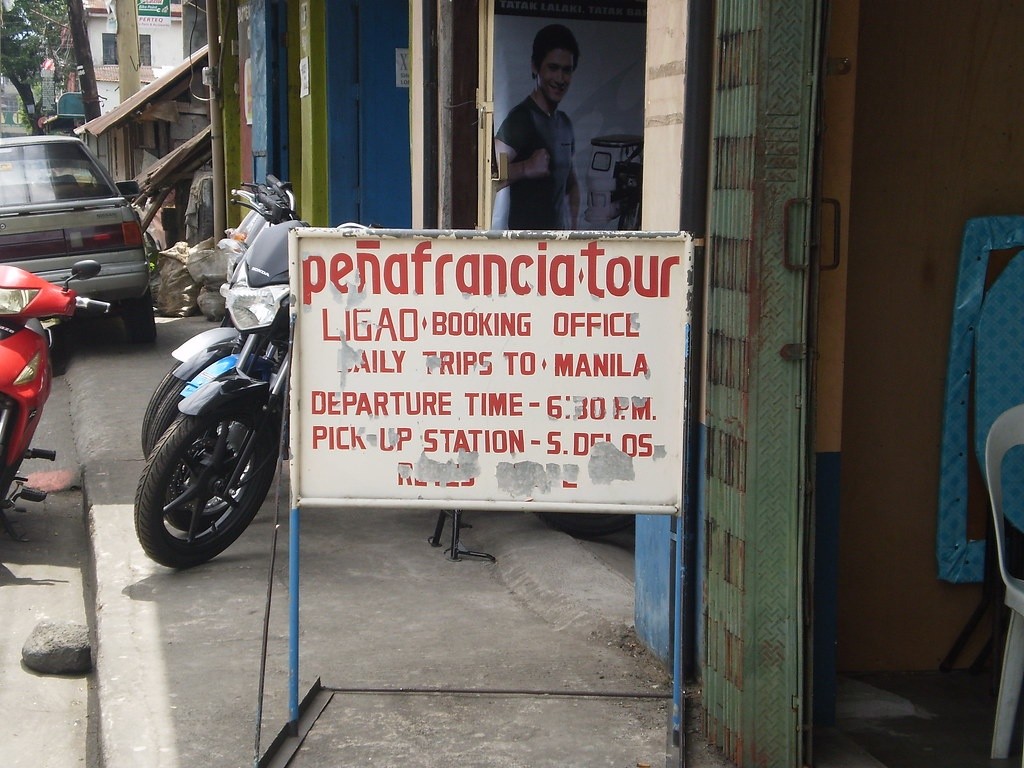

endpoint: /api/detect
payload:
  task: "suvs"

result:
[1,134,163,344]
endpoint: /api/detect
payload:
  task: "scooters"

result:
[119,177,645,573]
[0,259,113,520]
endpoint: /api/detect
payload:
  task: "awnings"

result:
[43,92,86,125]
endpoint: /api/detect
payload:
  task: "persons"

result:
[492,24,583,229]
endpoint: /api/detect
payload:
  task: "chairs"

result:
[38,174,79,198]
[986,400,1024,759]
